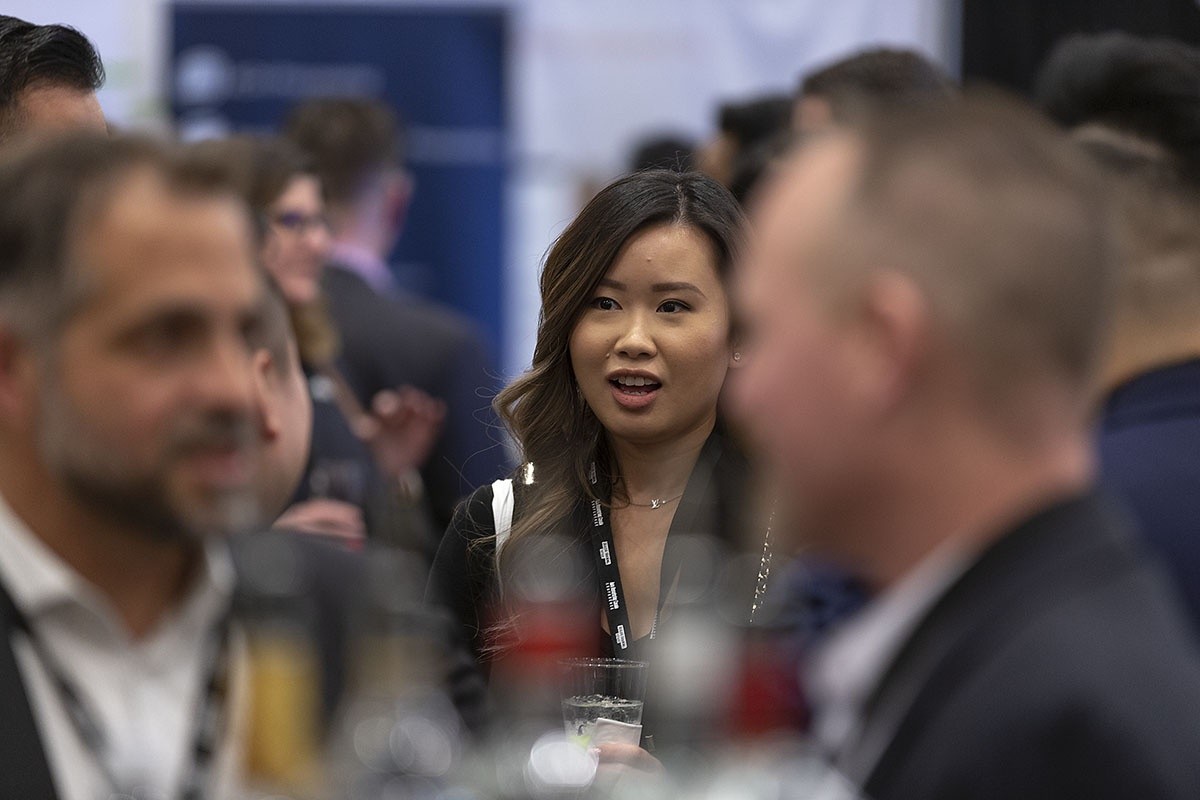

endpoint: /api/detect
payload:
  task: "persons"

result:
[0,15,1199,798]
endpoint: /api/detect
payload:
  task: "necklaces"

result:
[610,488,683,509]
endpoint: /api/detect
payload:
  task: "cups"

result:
[555,657,648,744]
